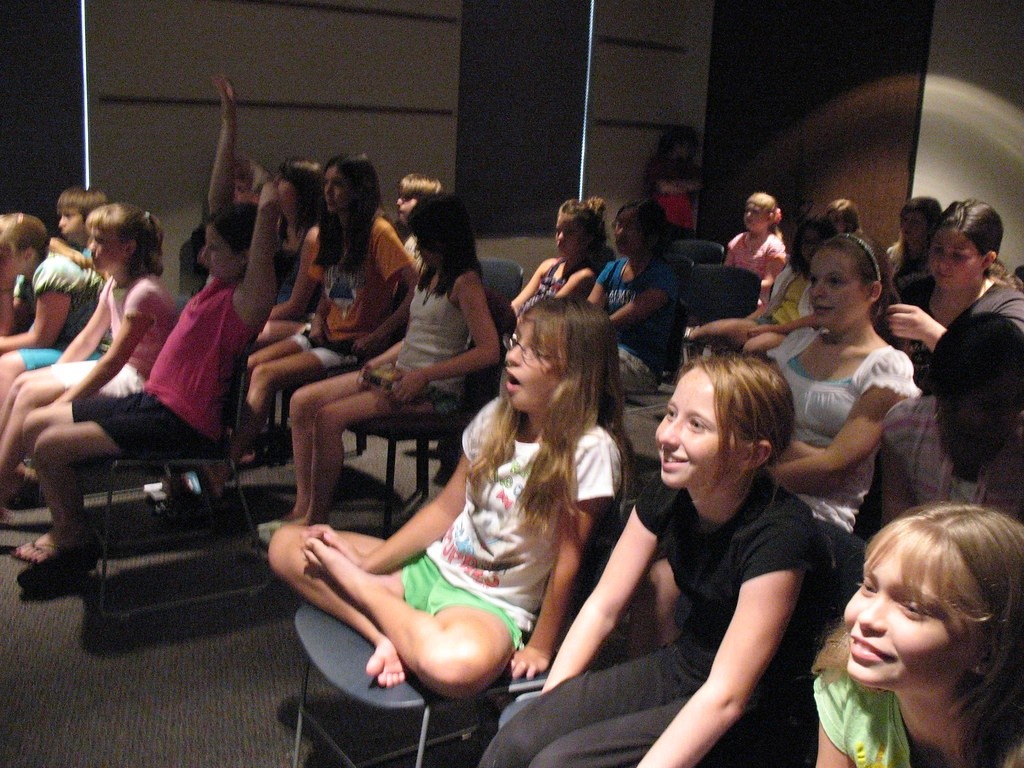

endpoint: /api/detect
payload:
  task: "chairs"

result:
[90,239,763,768]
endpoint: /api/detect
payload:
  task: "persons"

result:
[880,308,1024,531]
[885,199,1024,396]
[0,71,940,768]
[810,502,1024,768]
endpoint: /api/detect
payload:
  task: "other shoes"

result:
[256,519,332,547]
[11,467,43,507]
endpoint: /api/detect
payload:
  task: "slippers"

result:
[10,530,91,566]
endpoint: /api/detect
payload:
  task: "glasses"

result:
[502,332,552,360]
